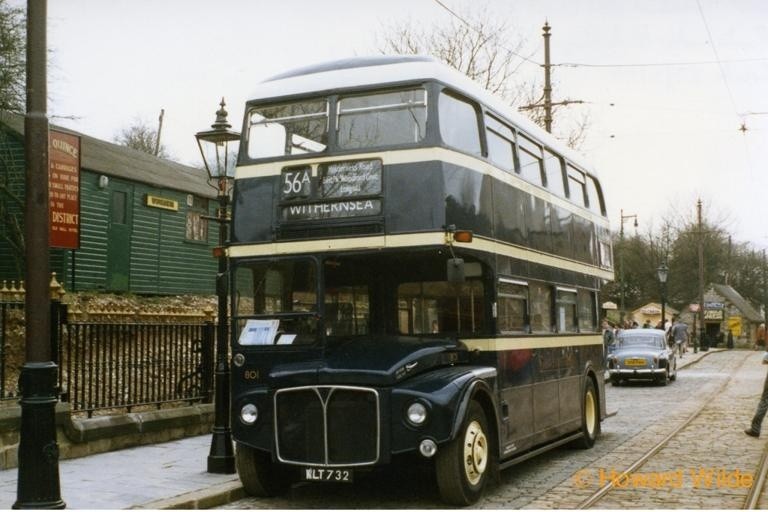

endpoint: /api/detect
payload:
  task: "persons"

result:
[753,322,765,352]
[601,316,691,362]
[742,352,768,438]
[431,321,438,333]
[726,328,734,348]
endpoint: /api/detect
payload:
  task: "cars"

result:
[608,329,677,387]
[643,319,690,355]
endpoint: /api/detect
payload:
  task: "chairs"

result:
[308,304,352,336]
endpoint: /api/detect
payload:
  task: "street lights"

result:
[619,212,638,328]
[196,96,243,475]
[655,263,669,332]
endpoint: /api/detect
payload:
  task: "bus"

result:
[228,54,620,505]
